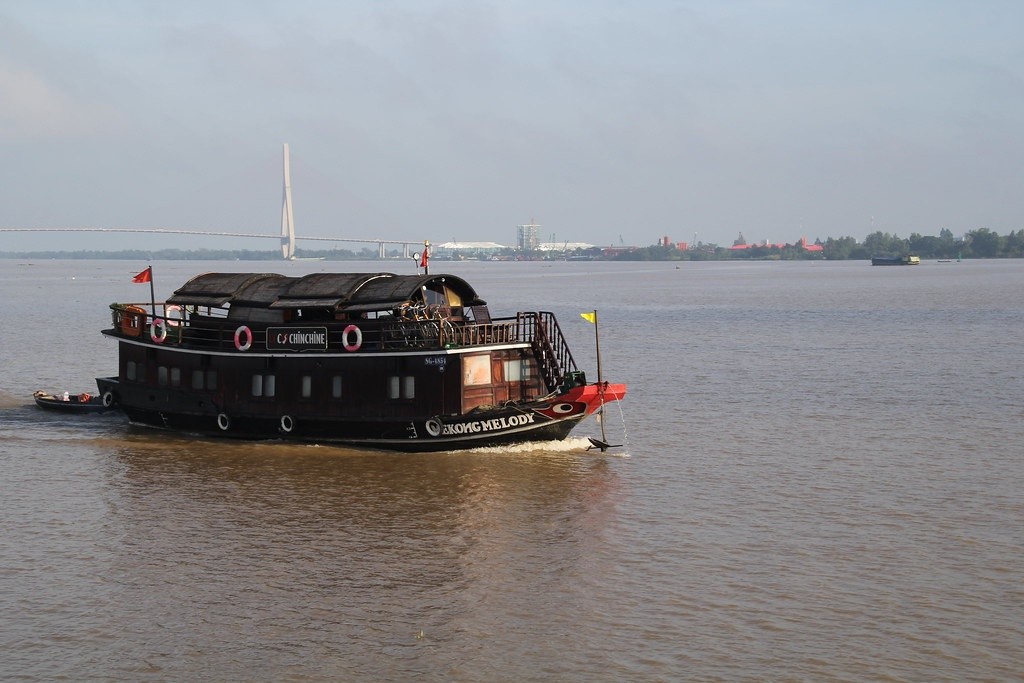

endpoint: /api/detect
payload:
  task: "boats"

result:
[292,256,326,262]
[102,269,627,451]
[938,260,952,262]
[34,392,107,412]
[870,255,921,265]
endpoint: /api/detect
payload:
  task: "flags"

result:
[420,248,427,267]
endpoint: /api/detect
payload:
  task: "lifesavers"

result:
[217,413,229,431]
[120,306,148,337]
[102,392,114,407]
[423,417,443,437]
[165,306,185,327]
[150,319,167,343]
[233,326,252,351]
[279,415,294,432]
[341,325,362,352]
